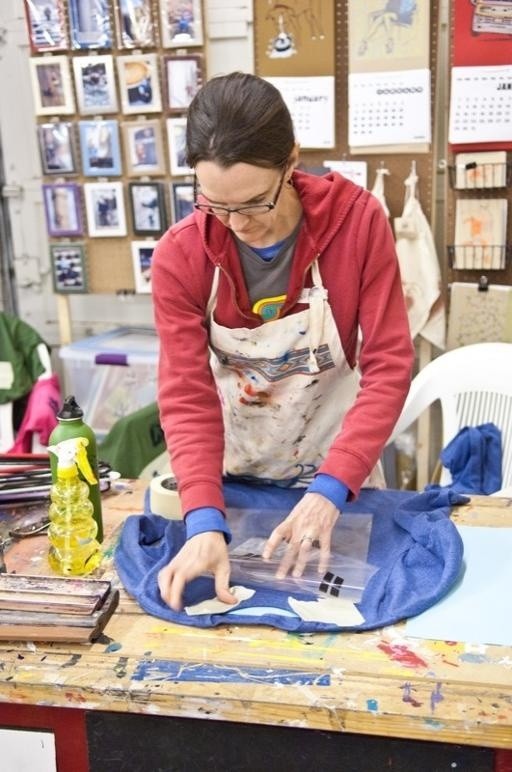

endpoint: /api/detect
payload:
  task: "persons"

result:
[357,0,417,56]
[148,71,418,613]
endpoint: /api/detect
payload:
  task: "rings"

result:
[300,535,315,544]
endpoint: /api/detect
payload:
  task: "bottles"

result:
[46,394,105,545]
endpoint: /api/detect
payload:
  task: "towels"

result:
[370,161,398,218]
[394,161,442,341]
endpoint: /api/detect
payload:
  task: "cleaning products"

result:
[48,438,102,575]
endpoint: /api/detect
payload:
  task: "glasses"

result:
[191,167,285,220]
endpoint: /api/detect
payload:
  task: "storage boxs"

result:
[59,326,161,444]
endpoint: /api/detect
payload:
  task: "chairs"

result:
[367,341,511,498]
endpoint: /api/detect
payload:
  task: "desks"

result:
[1,476,512,771]
